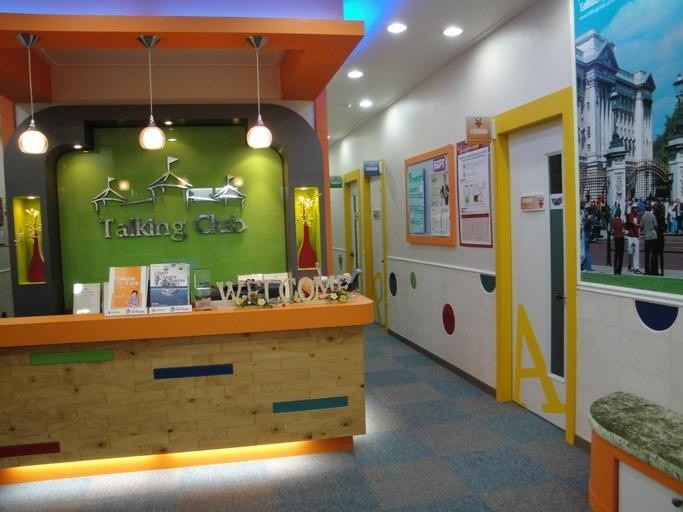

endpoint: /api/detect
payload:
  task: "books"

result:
[106,265,148,309]
[149,263,190,307]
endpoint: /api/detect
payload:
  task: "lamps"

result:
[246,35,273,149]
[14,33,48,155]
[138,35,165,150]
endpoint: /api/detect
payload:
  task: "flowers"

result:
[326,289,353,303]
[295,192,322,226]
[13,209,42,246]
[235,292,274,308]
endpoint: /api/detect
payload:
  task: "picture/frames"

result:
[569,0,683,308]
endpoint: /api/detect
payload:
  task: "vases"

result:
[299,228,317,268]
[28,238,44,282]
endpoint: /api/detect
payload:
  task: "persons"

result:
[126,289,138,307]
[579,186,682,278]
[438,173,448,205]
